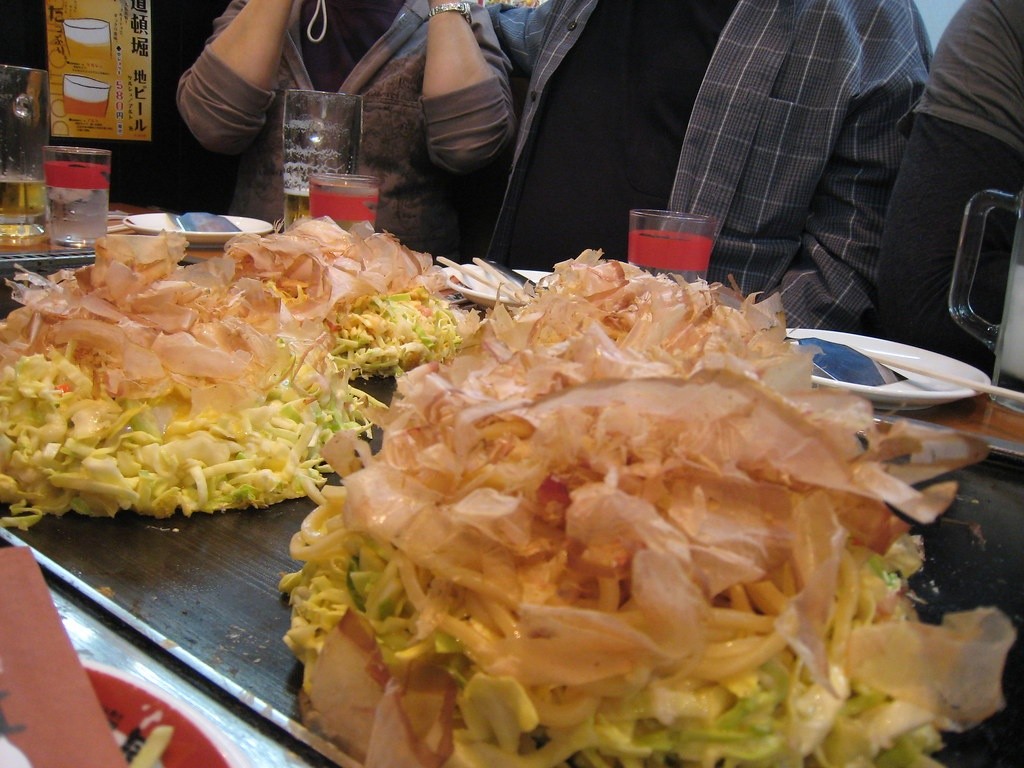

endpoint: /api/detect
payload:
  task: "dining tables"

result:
[0,195,1024,768]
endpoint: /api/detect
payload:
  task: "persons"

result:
[175,0,1024,381]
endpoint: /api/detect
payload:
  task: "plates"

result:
[123,212,273,248]
[447,270,553,310]
[783,328,991,411]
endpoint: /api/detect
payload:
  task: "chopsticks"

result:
[109,214,127,220]
[869,352,1024,403]
[436,256,527,302]
[108,223,129,234]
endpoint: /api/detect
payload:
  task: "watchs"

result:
[428,2,472,23]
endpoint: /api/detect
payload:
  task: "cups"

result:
[308,173,380,233]
[628,208,722,282]
[948,187,1024,418]
[0,63,51,244]
[283,90,364,232]
[43,145,110,251]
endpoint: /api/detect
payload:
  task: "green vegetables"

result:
[0,282,948,768]
